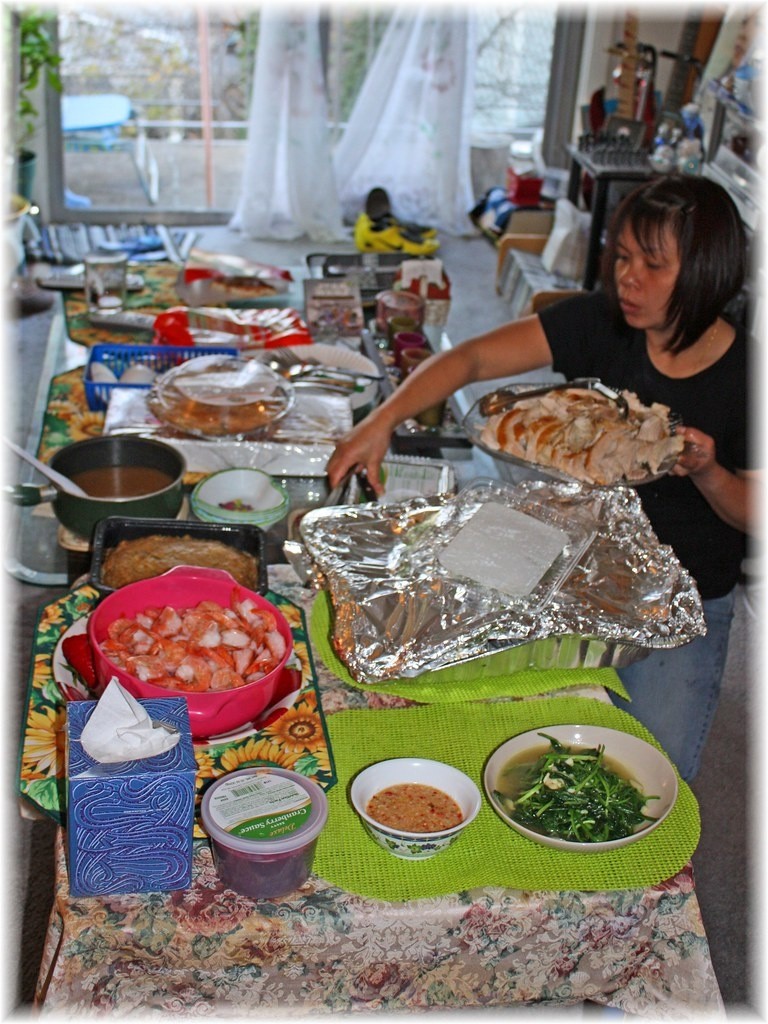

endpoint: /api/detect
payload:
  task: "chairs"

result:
[61,93,161,206]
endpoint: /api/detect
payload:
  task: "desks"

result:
[564,140,677,290]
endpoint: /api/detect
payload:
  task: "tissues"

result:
[62,674,198,899]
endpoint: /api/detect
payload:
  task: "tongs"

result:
[479,377,628,418]
[319,468,379,509]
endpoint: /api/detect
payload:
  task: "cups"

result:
[417,397,446,426]
[388,315,421,350]
[85,248,129,310]
[394,332,427,367]
[400,348,431,385]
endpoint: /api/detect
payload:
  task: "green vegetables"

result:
[491,732,664,843]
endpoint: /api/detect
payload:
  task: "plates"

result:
[53,610,302,746]
[462,383,684,488]
[484,724,678,852]
[254,346,378,410]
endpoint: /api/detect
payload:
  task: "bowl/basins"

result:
[147,361,294,442]
[87,566,293,737]
[87,516,268,608]
[351,758,481,861]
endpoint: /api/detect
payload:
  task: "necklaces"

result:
[647,315,720,372]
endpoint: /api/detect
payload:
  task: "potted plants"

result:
[13,10,65,200]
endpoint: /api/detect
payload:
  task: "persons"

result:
[325,173,765,785]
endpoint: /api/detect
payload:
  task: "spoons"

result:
[264,359,352,399]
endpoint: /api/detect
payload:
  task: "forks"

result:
[265,343,385,385]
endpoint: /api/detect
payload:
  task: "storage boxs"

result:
[495,211,553,264]
[495,249,581,317]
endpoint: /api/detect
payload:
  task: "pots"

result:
[5,435,186,544]
